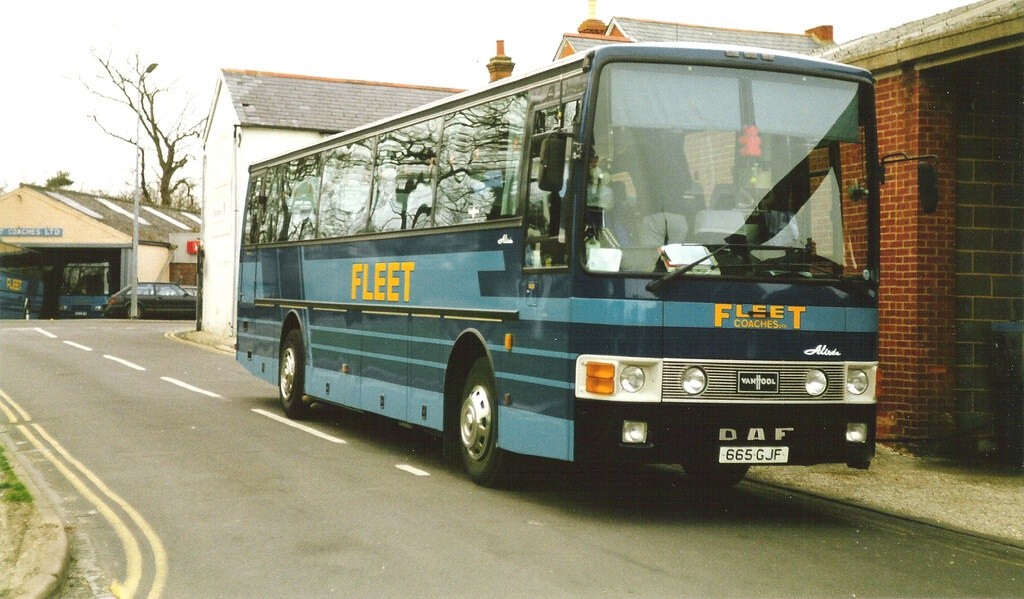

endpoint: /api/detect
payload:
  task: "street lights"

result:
[130,64,159,319]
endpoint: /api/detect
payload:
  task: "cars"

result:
[100,280,197,320]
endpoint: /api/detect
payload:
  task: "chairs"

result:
[605,161,801,248]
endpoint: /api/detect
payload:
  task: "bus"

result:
[54,259,112,318]
[0,239,46,322]
[232,39,881,512]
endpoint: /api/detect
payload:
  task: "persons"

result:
[23,294,31,321]
[586,146,798,243]
